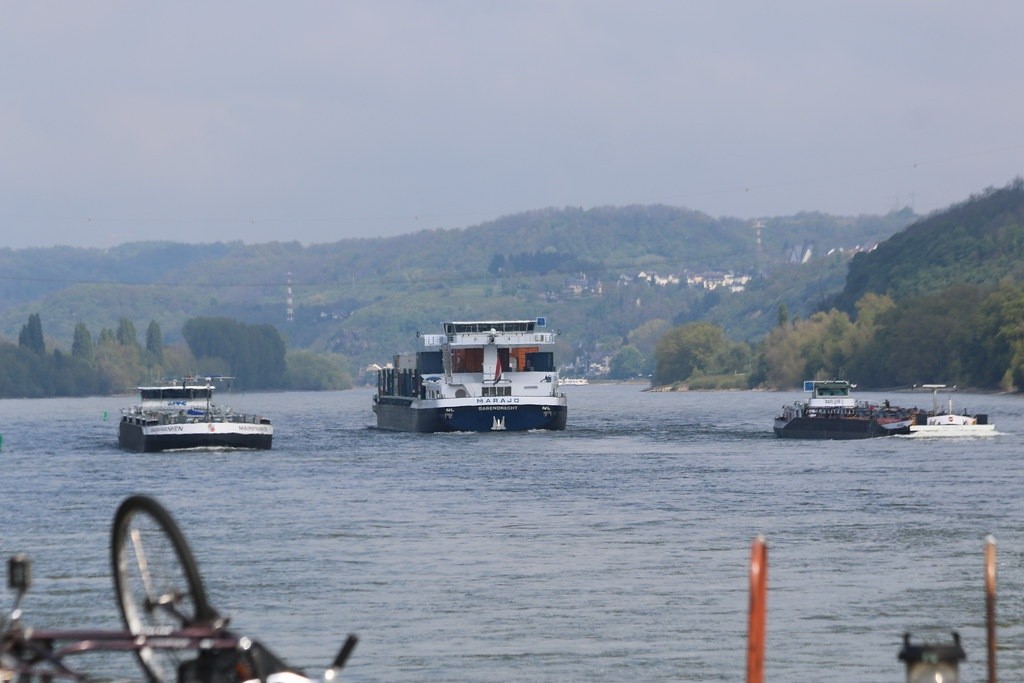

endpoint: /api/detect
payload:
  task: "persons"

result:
[882,399,890,407]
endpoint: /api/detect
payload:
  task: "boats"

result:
[883,397,996,439]
[361,315,568,434]
[116,367,274,451]
[771,370,995,439]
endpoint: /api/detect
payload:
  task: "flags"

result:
[493,356,502,385]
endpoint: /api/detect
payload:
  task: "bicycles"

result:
[0,492,360,683]
